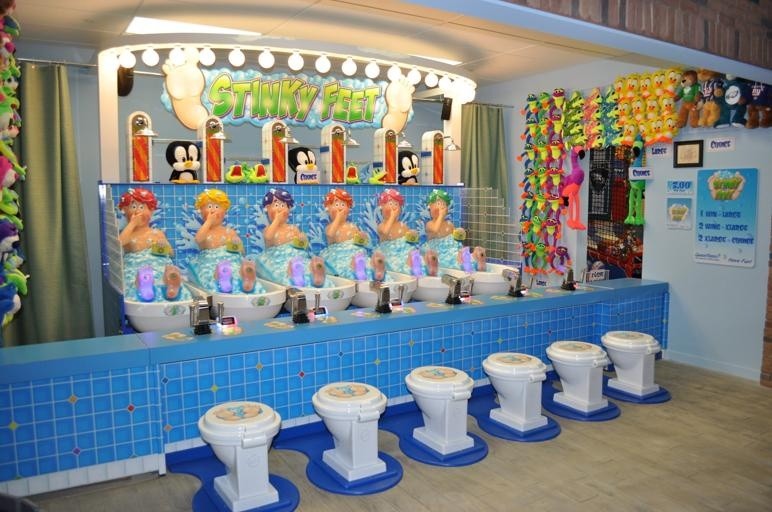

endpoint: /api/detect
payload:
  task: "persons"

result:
[261,187,325,288]
[321,187,386,281]
[426,188,486,274]
[115,187,181,301]
[376,188,438,277]
[194,188,257,293]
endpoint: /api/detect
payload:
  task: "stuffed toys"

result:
[368,166,387,185]
[165,141,201,184]
[398,150,421,185]
[0,0,30,330]
[515,66,772,274]
[288,147,322,184]
[345,161,362,185]
[225,161,268,184]
[515,67,772,275]
